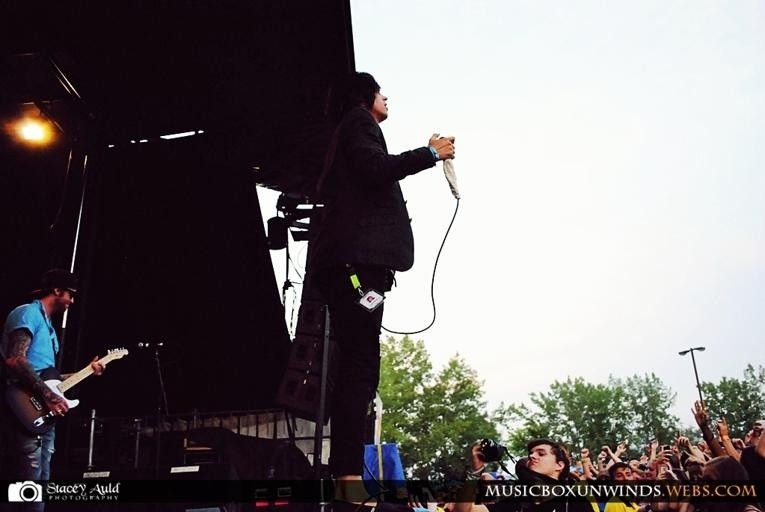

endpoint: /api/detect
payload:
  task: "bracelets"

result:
[426,144,440,162]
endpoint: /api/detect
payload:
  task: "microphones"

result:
[439,137,461,199]
[138,342,163,348]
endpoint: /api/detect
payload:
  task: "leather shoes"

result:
[334,499,415,512]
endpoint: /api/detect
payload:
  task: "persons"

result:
[406,399,765,511]
[311,72,456,512]
[2,267,107,512]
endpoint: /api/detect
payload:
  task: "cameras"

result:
[478,439,506,462]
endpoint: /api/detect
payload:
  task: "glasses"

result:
[67,288,77,298]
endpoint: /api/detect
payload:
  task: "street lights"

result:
[676,345,705,411]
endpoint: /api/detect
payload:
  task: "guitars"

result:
[9,347,128,435]
